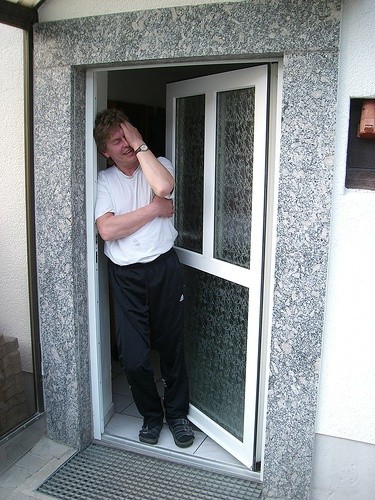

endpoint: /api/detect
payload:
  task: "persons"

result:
[93,108,195,448]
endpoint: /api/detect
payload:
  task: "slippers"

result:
[139,419,163,444]
[169,419,195,447]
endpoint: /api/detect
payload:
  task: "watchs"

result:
[133,144,151,155]
